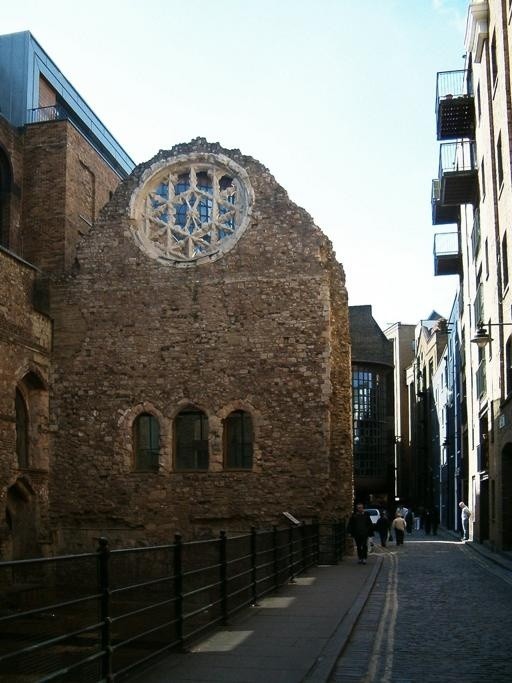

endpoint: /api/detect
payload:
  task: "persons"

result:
[375,513,391,547]
[459,502,471,540]
[423,512,432,535]
[392,512,407,546]
[405,511,413,536]
[430,507,440,536]
[347,503,375,566]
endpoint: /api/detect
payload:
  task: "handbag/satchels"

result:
[389,536,393,541]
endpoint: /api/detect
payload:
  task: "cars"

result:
[364,508,381,524]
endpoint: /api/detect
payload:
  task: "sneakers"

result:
[409,533,411,536]
[358,559,367,564]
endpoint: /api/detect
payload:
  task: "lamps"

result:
[467,319,512,349]
